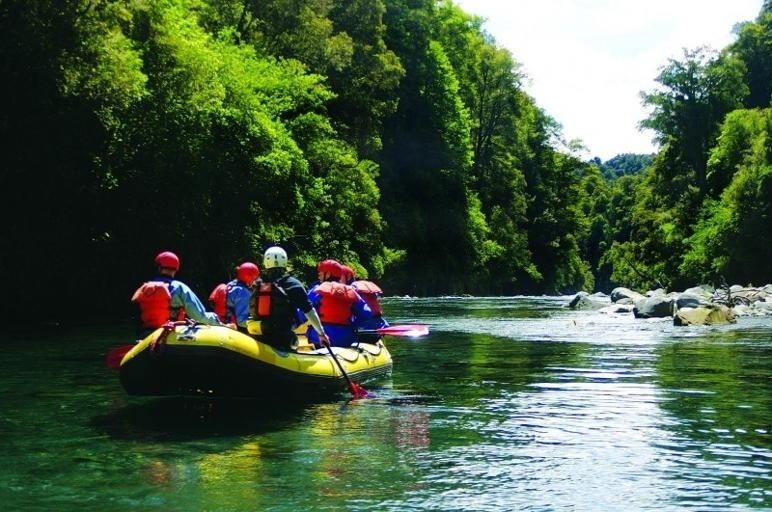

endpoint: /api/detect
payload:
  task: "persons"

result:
[132,246,388,353]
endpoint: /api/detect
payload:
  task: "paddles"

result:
[108,344,134,368]
[324,340,367,400]
[355,324,429,338]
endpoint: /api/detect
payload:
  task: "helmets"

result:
[340,264,355,283]
[262,245,289,269]
[318,258,343,279]
[237,261,261,285]
[154,250,181,271]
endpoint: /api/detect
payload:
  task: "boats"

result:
[119,318,393,399]
